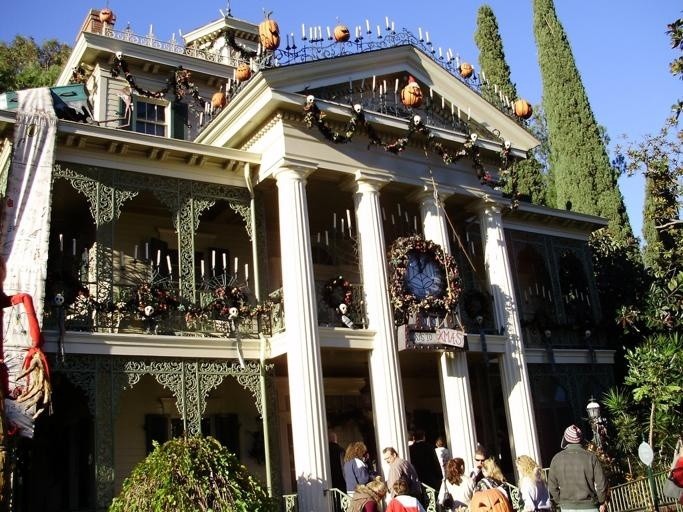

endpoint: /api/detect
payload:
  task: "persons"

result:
[343,439,377,512]
[406,430,551,511]
[546,425,606,511]
[382,446,422,494]
[383,479,425,511]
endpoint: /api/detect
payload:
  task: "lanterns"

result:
[98,8,113,24]
[458,63,472,79]
[514,98,532,120]
[210,93,224,108]
[233,63,250,82]
[258,19,280,52]
[334,26,350,42]
[400,82,421,107]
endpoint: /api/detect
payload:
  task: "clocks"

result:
[406,253,445,301]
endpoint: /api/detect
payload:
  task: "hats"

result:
[560,425,584,449]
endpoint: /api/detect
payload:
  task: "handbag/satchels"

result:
[443,492,454,508]
[504,481,525,510]
